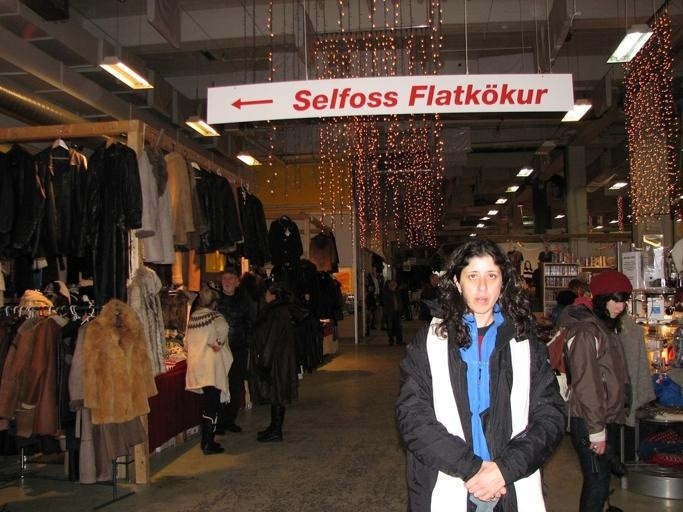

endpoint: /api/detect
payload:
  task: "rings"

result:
[594,446,597,449]
[491,497,495,500]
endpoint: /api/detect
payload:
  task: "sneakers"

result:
[227,422,243,433]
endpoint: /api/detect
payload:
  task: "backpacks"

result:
[546,328,603,402]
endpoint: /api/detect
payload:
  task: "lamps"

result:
[465,165,535,238]
[186,61,221,137]
[561,41,592,123]
[236,153,262,166]
[606,0,654,64]
[97,1,155,92]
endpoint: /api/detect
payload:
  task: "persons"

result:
[251,283,295,443]
[366,244,587,345]
[564,270,633,512]
[184,288,233,455]
[393,239,568,512]
[190,267,257,436]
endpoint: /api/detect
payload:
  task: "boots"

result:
[257,405,285,442]
[200,414,225,455]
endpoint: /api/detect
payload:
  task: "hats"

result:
[589,271,633,297]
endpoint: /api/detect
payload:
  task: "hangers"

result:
[0,301,97,326]
[49,129,71,160]
[105,135,116,150]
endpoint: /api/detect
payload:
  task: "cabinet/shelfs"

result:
[632,287,680,375]
[538,262,614,313]
